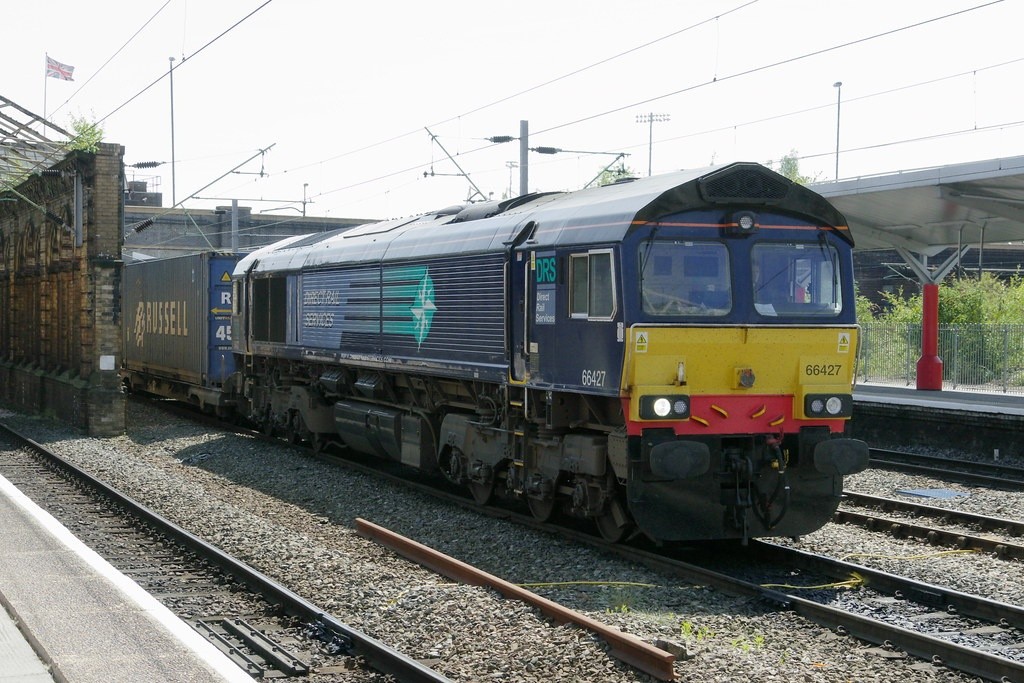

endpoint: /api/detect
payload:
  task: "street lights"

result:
[169,56,177,207]
[832,82,842,185]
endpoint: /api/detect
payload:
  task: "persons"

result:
[727,260,767,309]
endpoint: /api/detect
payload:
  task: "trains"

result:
[110,158,872,548]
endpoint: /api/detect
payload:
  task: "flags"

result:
[47,56,75,81]
